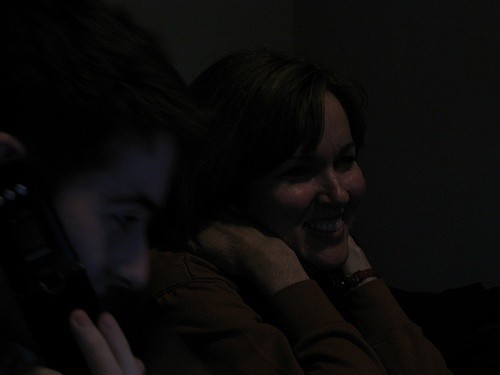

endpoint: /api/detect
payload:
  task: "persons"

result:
[113,48,453,375]
[0,0,213,375]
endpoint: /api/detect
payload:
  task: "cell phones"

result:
[0,160,103,375]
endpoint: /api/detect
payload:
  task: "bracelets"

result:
[330,268,382,298]
